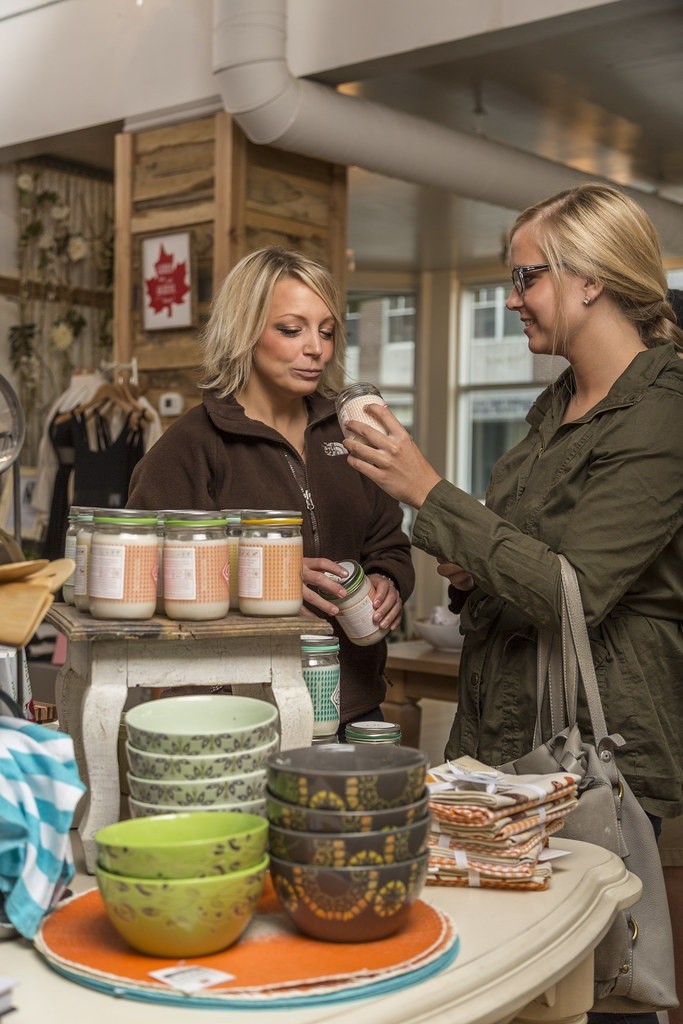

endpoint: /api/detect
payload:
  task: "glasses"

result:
[511,261,566,297]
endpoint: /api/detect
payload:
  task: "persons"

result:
[342,182,683,1024]
[123,249,416,743]
[31,367,111,602]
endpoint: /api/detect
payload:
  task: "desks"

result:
[0,838,644,1024]
[379,640,460,753]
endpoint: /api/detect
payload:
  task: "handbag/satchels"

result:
[470,553,681,1014]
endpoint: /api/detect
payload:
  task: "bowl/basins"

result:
[93,695,431,957]
[413,616,465,653]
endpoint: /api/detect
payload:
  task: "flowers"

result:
[12,163,114,466]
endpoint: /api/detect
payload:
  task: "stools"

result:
[47,604,335,877]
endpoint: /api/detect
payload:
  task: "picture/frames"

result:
[134,230,199,331]
